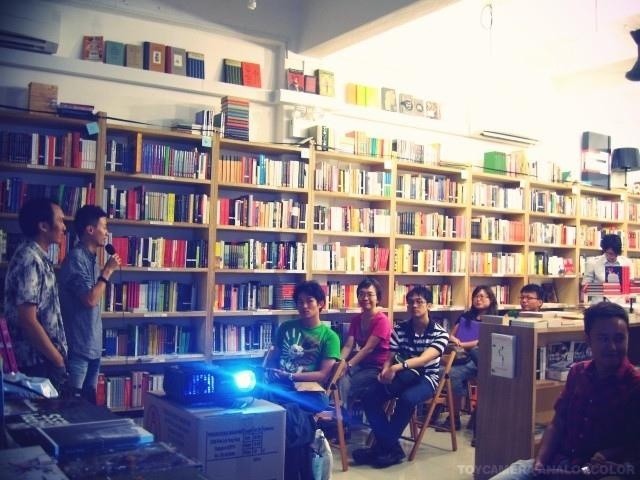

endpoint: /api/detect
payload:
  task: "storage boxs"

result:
[141,388,287,480]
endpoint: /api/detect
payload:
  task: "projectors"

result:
[163,362,258,406]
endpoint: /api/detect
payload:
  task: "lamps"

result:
[610,147,640,190]
[247,0,257,10]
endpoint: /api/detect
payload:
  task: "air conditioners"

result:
[0,0,62,55]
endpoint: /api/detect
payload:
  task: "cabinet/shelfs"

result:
[393,158,470,326]
[0,108,107,279]
[310,151,393,327]
[626,191,640,280]
[579,182,626,278]
[469,169,529,312]
[472,321,640,480]
[198,137,310,363]
[528,177,579,311]
[95,118,221,424]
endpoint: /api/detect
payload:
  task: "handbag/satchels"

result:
[310,428,333,479]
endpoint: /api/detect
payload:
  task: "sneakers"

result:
[352,447,378,466]
[376,445,406,469]
[436,417,462,432]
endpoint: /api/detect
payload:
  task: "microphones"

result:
[105,244,115,255]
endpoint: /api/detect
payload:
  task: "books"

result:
[0,35,640,479]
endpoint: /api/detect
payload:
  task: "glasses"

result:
[518,296,541,301]
[406,299,428,307]
[357,292,376,300]
[605,248,616,256]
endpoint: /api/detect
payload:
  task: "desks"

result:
[1,383,216,480]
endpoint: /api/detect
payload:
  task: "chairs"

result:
[311,358,356,472]
[365,350,457,461]
